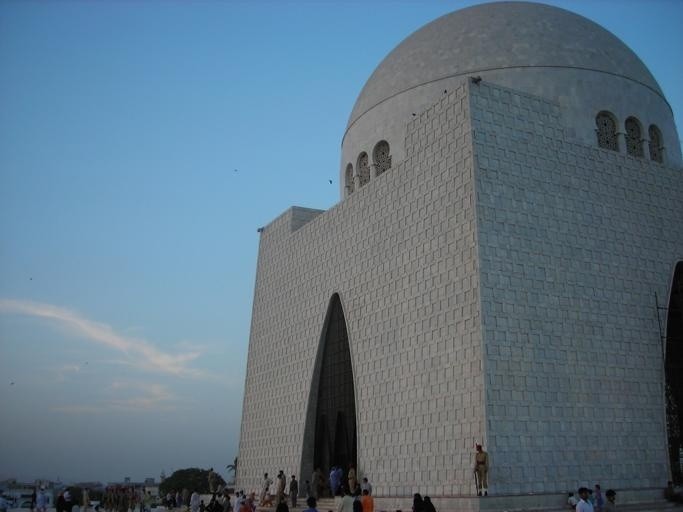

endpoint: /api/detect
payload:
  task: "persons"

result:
[565,483,616,512]
[412,493,435,511]
[1,478,154,511]
[472,442,489,496]
[160,463,374,511]
[662,481,682,506]
